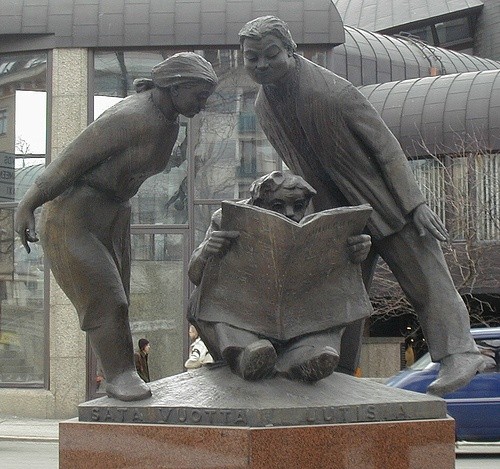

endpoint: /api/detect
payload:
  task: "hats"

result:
[138,338,149,350]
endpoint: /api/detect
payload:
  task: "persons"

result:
[14,49,218,401]
[240,17,489,395]
[95,369,105,392]
[185,325,214,373]
[133,337,152,384]
[184,171,346,384]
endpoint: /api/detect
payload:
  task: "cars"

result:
[381,327,500,443]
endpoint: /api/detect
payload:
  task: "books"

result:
[198,200,375,343]
